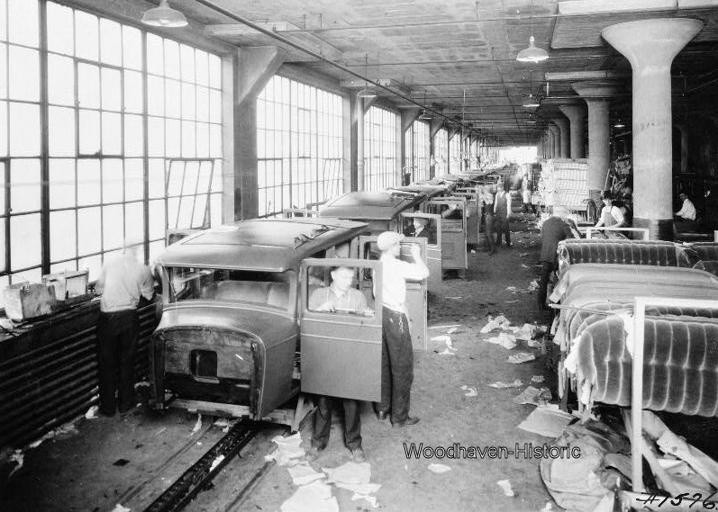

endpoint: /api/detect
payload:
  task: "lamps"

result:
[517,0,550,62]
[141,0,188,28]
[614,118,625,128]
[356,53,376,97]
[527,113,536,123]
[522,68,540,107]
[419,90,431,120]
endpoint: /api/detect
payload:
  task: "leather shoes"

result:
[377,409,419,429]
[303,444,324,463]
[351,446,367,463]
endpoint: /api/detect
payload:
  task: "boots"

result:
[474,235,497,255]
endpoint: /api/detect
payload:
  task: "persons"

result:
[92,244,154,418]
[475,166,535,256]
[371,229,431,429]
[305,264,375,462]
[672,189,698,232]
[538,204,574,310]
[442,201,462,280]
[594,191,625,239]
[408,210,433,238]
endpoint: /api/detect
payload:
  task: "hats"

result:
[376,229,406,251]
[599,189,616,201]
